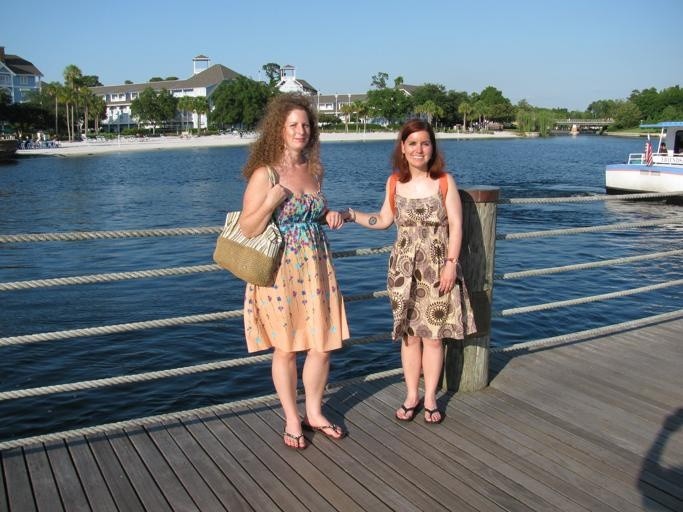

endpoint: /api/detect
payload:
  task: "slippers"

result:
[396,405,443,424]
[282,422,346,449]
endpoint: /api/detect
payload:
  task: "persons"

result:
[24,137,31,149]
[660,143,667,155]
[236,90,344,449]
[342,119,477,425]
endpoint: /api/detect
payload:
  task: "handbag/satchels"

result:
[212,211,283,286]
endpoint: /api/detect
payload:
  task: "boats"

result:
[605,122,683,194]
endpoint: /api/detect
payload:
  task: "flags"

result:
[645,134,652,167]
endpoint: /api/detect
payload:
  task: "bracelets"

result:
[443,257,458,264]
[353,210,356,221]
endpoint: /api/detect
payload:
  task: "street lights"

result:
[317,90,351,125]
